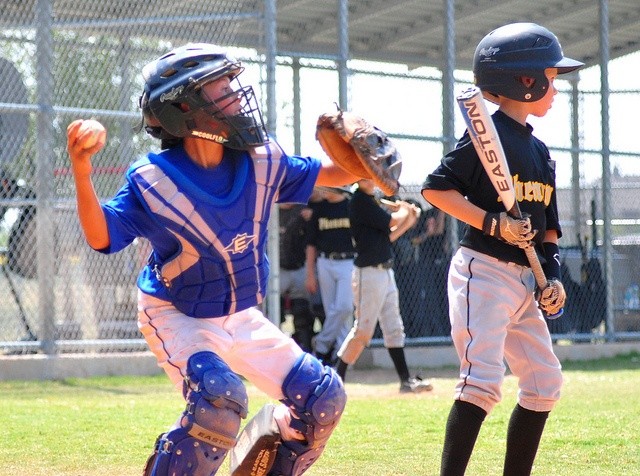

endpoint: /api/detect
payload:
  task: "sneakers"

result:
[400,377,432,393]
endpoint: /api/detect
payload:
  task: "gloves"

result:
[535,279,567,315]
[482,211,540,249]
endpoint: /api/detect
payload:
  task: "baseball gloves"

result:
[315,101,403,197]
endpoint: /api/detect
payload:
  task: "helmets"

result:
[136,42,273,152]
[472,22,586,106]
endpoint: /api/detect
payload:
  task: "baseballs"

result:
[77,120,106,149]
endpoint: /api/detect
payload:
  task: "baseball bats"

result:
[456,86,564,320]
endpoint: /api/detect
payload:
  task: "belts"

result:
[326,252,354,260]
[372,262,394,270]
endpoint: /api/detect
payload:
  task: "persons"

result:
[66,42,404,475]
[393,197,434,338]
[419,21,587,476]
[332,180,434,394]
[547,258,579,343]
[574,258,607,343]
[279,202,325,353]
[305,186,356,368]
[420,205,453,338]
